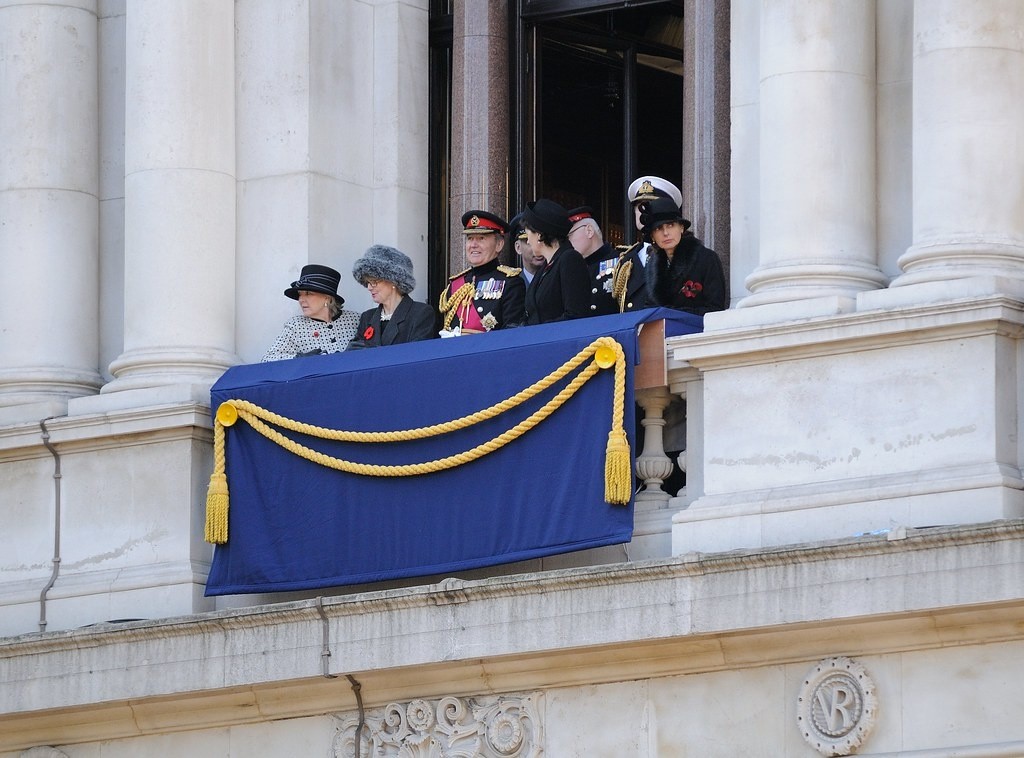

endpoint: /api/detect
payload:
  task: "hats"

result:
[523,199,573,236]
[461,210,512,238]
[567,206,594,225]
[284,265,345,304]
[638,197,691,243]
[509,212,528,240]
[352,244,416,295]
[628,176,683,210]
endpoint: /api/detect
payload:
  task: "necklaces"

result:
[381,308,393,321]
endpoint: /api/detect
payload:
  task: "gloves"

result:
[438,325,461,339]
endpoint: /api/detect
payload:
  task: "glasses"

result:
[363,279,383,287]
[567,224,587,238]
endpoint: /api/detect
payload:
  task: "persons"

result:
[434,210,526,340]
[260,265,363,364]
[345,245,436,353]
[518,199,591,326]
[568,175,728,318]
[510,212,546,289]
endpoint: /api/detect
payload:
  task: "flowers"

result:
[679,280,702,297]
